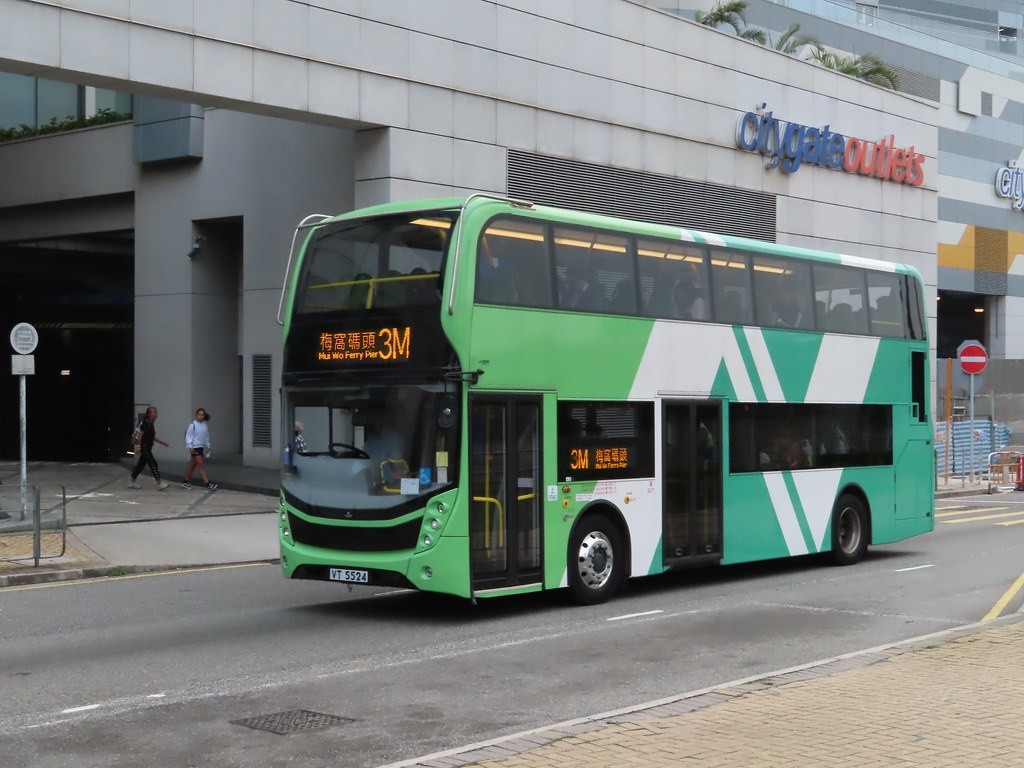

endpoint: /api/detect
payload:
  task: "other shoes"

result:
[128,482,142,488]
[157,482,170,491]
[274,505,278,512]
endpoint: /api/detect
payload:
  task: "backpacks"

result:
[184,423,195,445]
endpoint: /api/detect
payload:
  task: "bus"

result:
[269,192,937,606]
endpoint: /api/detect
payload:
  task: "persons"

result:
[179,408,218,490]
[128,406,170,491]
[295,421,308,452]
[354,416,850,545]
[440,230,834,333]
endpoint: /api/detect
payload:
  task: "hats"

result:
[778,273,807,290]
[295,422,303,431]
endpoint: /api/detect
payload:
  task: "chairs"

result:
[378,270,402,306]
[348,272,375,306]
[717,290,743,320]
[831,303,853,332]
[406,267,434,304]
[805,301,826,330]
[649,285,678,316]
[480,265,524,305]
[611,277,645,314]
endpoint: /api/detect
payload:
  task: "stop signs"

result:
[959,343,989,376]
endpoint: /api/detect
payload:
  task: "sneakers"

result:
[206,483,217,490]
[181,479,192,489]
